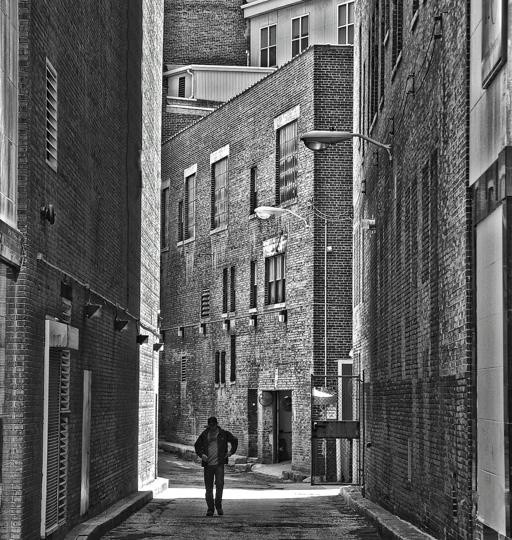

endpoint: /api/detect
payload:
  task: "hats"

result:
[208,417,217,425]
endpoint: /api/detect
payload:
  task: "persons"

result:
[195,417,238,516]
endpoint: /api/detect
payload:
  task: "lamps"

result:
[301,130,394,162]
[254,205,309,229]
[83,291,165,353]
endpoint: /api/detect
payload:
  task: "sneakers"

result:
[206,506,223,516]
